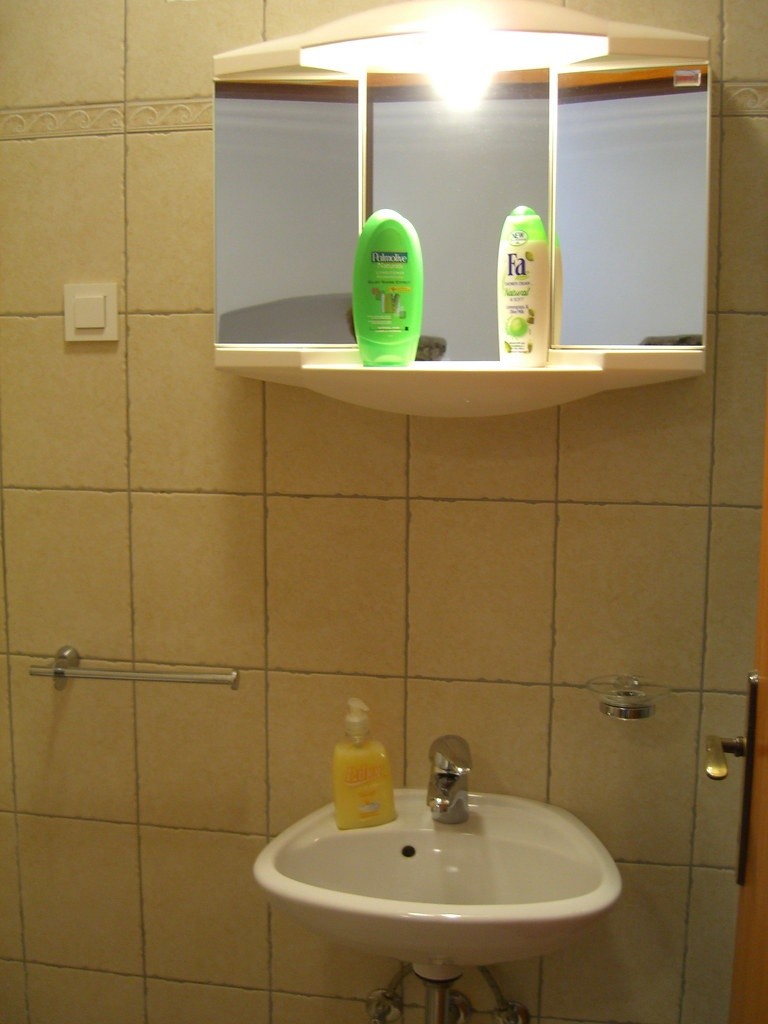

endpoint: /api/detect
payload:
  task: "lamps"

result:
[298,0,610,113]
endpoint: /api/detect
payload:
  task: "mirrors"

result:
[210,21,710,418]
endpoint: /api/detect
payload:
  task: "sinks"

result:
[253,789,622,963]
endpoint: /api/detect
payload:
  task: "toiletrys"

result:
[495,205,551,367]
[331,697,396,830]
[352,207,423,367]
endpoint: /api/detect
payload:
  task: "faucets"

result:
[427,733,470,822]
